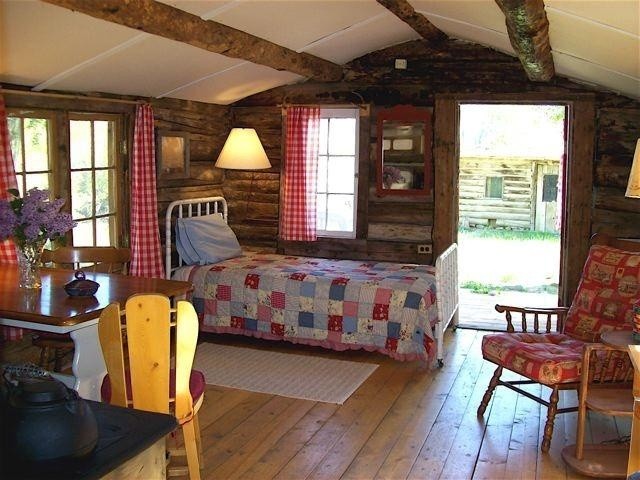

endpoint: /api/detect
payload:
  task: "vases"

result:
[384,183,393,190]
[13,238,50,288]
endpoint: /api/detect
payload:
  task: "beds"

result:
[166,196,459,368]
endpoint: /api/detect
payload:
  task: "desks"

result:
[0,262,194,403]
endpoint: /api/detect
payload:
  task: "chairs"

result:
[98,293,207,480]
[477,232,640,453]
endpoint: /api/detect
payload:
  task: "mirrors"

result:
[376,105,431,197]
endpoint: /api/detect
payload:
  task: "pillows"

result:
[175,214,242,265]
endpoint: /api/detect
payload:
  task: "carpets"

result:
[193,341,379,405]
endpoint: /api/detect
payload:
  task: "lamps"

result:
[625,138,640,198]
[214,128,272,170]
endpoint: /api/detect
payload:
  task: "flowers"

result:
[0,187,78,273]
[382,164,403,186]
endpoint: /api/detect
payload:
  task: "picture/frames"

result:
[156,131,190,181]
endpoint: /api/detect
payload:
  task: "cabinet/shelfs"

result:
[561,330,640,480]
[627,344,640,480]
[0,401,179,480]
